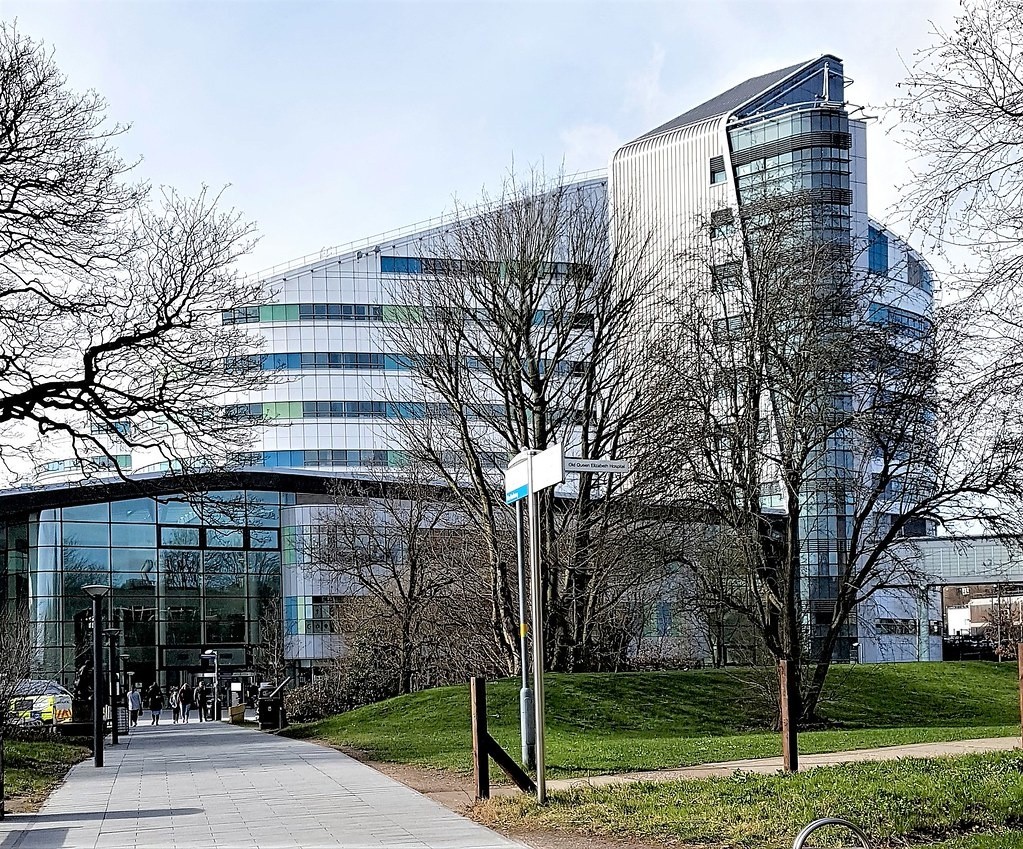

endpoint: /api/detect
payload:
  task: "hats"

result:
[199,681,205,686]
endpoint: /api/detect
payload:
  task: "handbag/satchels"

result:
[139,705,143,716]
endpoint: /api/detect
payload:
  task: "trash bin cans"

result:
[258,687,282,730]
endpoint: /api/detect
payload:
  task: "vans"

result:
[3,679,74,725]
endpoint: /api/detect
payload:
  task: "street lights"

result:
[119,653,130,708]
[80,584,111,768]
[103,628,123,745]
[127,671,135,692]
[992,583,1001,663]
[204,649,218,721]
[507,445,544,771]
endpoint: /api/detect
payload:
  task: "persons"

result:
[127,685,141,727]
[147,681,207,725]
